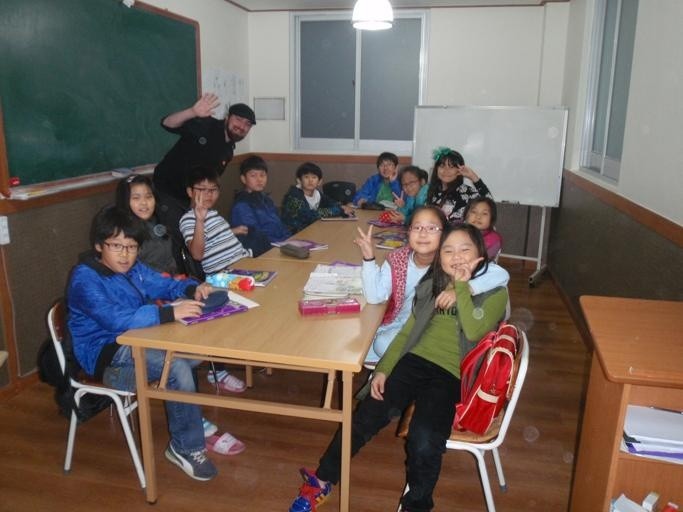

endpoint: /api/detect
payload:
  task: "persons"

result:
[421,149,494,225]
[386,165,430,228]
[229,154,293,257]
[348,151,403,212]
[351,204,510,360]
[114,172,246,438]
[278,161,356,232]
[152,89,257,207]
[63,202,216,482]
[288,219,510,511]
[176,166,250,275]
[458,195,502,262]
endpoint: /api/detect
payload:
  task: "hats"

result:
[229,103,256,124]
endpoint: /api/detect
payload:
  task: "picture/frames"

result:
[253,96,285,121]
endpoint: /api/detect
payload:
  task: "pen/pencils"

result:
[162,300,184,308]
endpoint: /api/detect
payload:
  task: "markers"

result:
[501,200,520,205]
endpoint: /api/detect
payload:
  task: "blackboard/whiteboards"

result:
[0,0,202,198]
[411,105,568,208]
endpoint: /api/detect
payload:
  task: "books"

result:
[318,216,359,222]
[299,263,364,295]
[326,260,362,267]
[268,237,329,252]
[373,237,403,251]
[208,267,278,288]
[156,288,261,327]
[618,404,683,467]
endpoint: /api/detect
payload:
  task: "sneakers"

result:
[289,468,332,512]
[164,439,217,481]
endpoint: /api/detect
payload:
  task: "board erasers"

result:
[111,167,131,178]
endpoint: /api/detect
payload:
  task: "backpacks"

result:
[453,321,520,435]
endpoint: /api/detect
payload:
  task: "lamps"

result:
[351,1,392,30]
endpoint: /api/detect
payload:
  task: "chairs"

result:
[44,298,159,489]
[395,330,528,511]
[176,236,207,279]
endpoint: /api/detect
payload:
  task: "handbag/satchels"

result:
[36,337,110,423]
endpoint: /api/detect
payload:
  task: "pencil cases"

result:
[298,298,360,316]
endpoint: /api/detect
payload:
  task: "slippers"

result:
[204,432,246,456]
[211,375,247,393]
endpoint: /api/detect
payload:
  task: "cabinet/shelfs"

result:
[565,294,682,510]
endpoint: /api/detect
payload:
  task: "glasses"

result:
[193,187,220,195]
[409,224,442,233]
[103,241,140,253]
[401,180,418,188]
